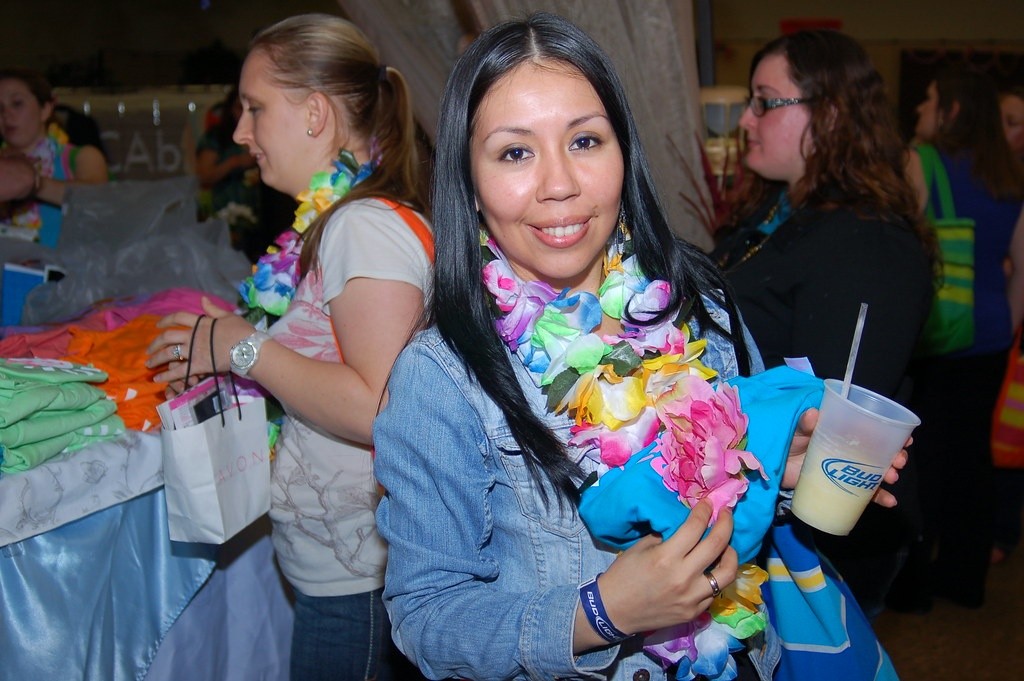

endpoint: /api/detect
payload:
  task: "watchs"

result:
[229,330,273,382]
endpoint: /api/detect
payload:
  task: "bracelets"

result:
[577,572,637,644]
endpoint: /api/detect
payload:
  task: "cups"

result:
[790,378,921,536]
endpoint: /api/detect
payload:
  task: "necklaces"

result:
[238,149,384,462]
[472,221,771,526]
[1,124,69,233]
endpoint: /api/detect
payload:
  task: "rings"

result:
[705,572,720,597]
[173,345,182,360]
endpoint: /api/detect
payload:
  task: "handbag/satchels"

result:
[159,314,272,546]
[914,145,977,347]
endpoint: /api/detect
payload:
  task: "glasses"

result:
[744,94,817,117]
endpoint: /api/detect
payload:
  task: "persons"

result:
[180,83,299,263]
[704,28,1024,635]
[0,69,109,253]
[147,14,438,680]
[372,15,912,681]
